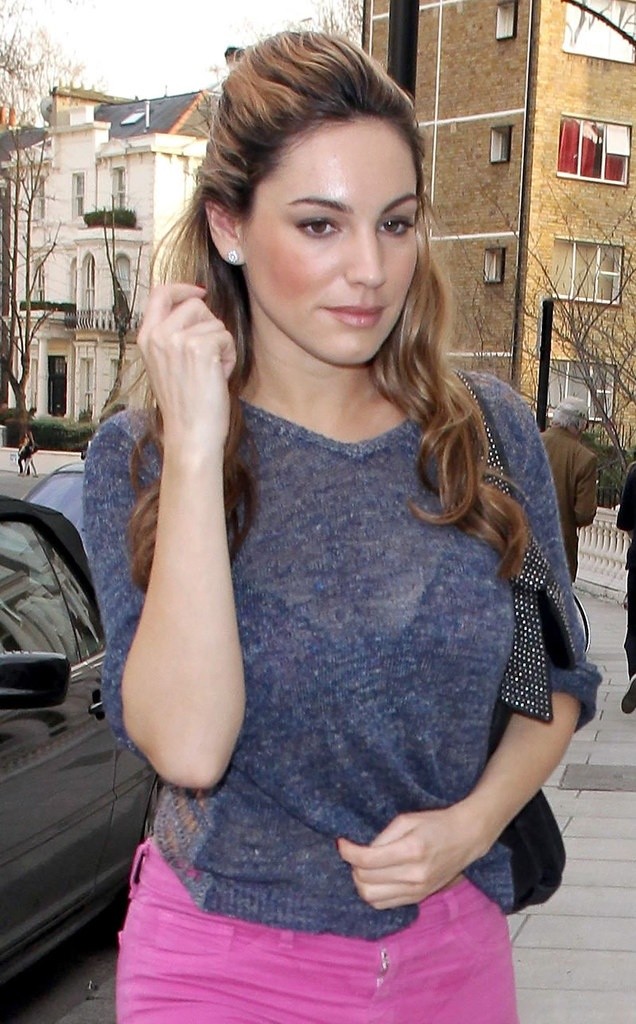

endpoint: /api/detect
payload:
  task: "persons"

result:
[539,396,598,582]
[84,32,602,1024]
[17,425,38,476]
[616,462,636,713]
[81,430,95,460]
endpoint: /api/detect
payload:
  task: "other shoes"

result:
[621,674,636,714]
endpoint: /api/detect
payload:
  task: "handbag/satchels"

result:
[453,369,576,908]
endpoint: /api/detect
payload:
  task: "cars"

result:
[0,456,162,987]
